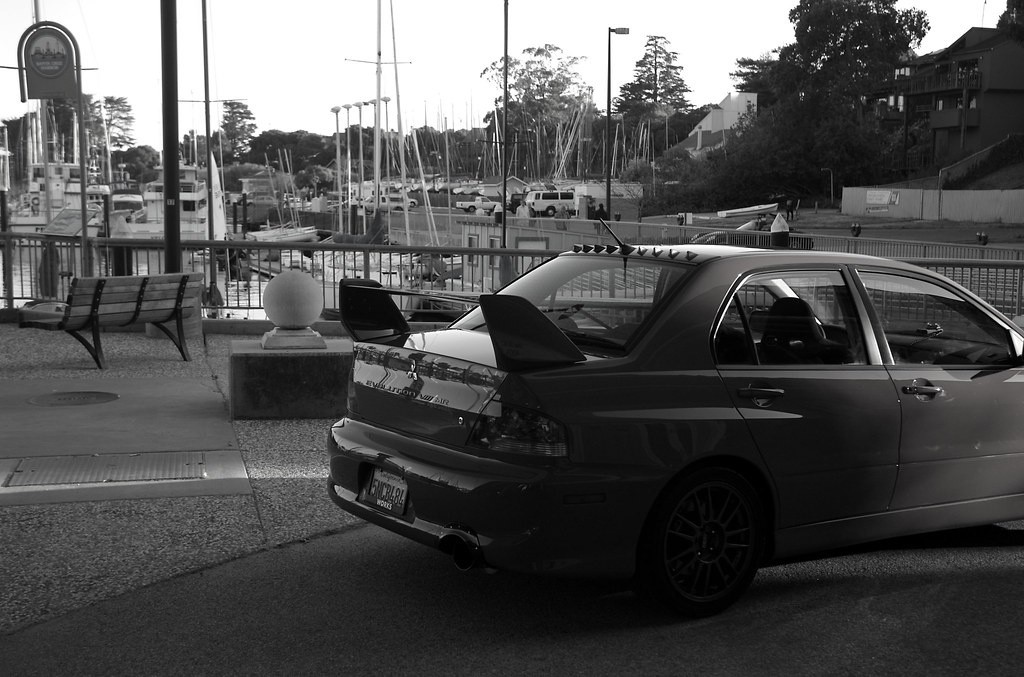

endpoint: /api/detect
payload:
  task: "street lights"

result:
[822,166,835,206]
[606,27,631,221]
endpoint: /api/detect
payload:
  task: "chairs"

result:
[19,271,204,370]
[716,329,753,364]
[760,297,840,366]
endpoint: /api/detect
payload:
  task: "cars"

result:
[390,194,418,208]
[360,195,411,214]
[284,193,302,203]
[323,242,1023,606]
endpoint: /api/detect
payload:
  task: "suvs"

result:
[510,193,527,214]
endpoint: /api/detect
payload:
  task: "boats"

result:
[717,202,779,218]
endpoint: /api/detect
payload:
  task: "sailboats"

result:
[121,6,471,314]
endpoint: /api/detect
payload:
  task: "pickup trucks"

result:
[456,195,500,213]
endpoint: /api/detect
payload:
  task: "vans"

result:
[523,191,576,216]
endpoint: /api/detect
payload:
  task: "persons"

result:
[555,206,570,231]
[516,201,536,228]
[593,203,607,234]
